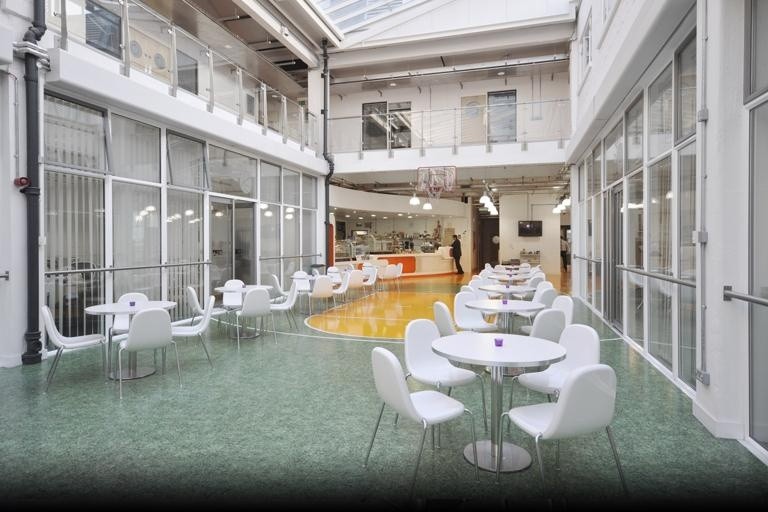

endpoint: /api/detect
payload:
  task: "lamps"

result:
[478,190,489,203]
[423,198,432,209]
[409,193,420,205]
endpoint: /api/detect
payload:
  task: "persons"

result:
[450,234,464,275]
[560,235,571,273]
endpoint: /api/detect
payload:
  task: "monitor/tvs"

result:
[518,221,542,236]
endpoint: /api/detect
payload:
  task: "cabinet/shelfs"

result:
[519,253,540,269]
